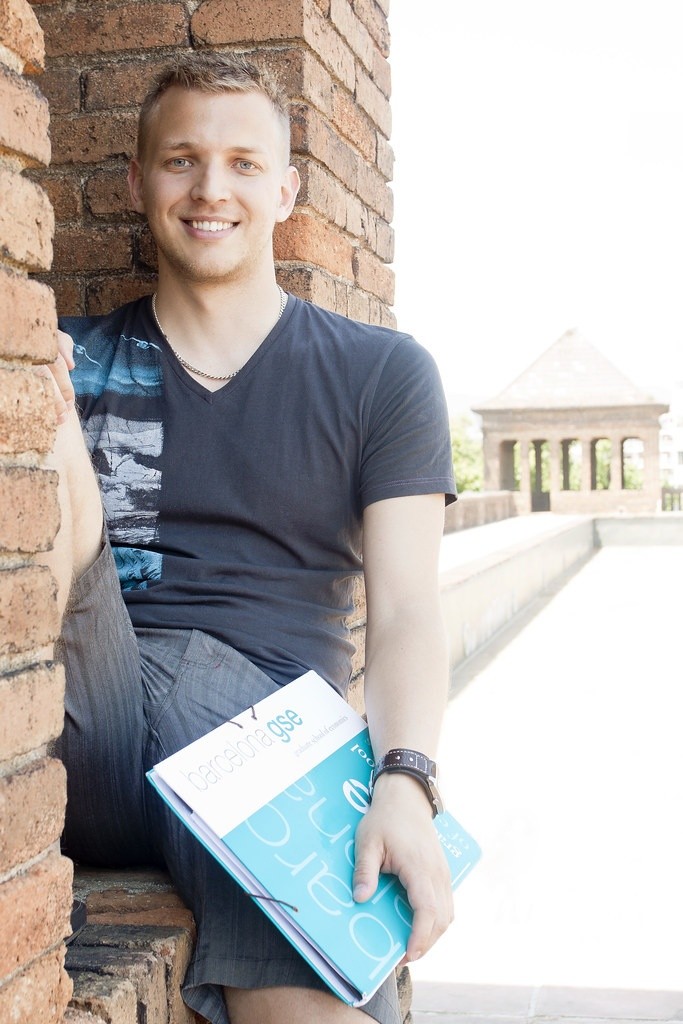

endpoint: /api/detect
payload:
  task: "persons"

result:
[38,52,459,1024]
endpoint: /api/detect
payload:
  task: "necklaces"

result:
[152,283,286,379]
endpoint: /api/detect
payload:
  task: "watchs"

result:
[369,748,445,819]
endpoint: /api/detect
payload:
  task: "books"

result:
[146,668,488,1009]
